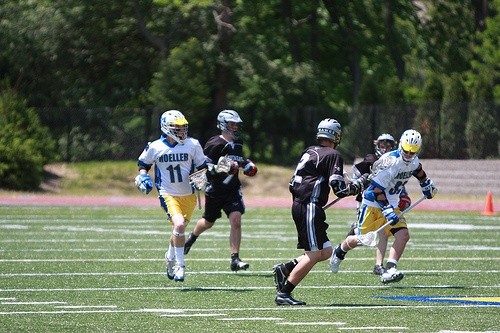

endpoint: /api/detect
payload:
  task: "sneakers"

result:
[329,245,343,274]
[230,258,249,271]
[275,291,306,305]
[381,267,404,283]
[273,263,290,293]
[373,264,386,274]
[172,261,186,282]
[184,233,194,255]
[165,251,176,280]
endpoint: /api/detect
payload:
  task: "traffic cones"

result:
[481,191,496,216]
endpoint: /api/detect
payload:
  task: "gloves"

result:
[355,173,370,189]
[227,160,239,174]
[420,179,438,199]
[243,159,257,177]
[134,174,153,194]
[398,194,411,212]
[380,205,399,226]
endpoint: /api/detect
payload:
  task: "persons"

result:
[345,134,410,275]
[328,129,437,282]
[272,118,372,305]
[135,110,208,281]
[184,110,257,271]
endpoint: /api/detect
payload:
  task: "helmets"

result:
[160,108,189,145]
[398,129,422,165]
[217,110,243,140]
[316,118,342,145]
[374,134,396,159]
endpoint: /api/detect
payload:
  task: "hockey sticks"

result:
[188,167,209,209]
[214,156,250,174]
[354,194,427,248]
[322,155,399,210]
